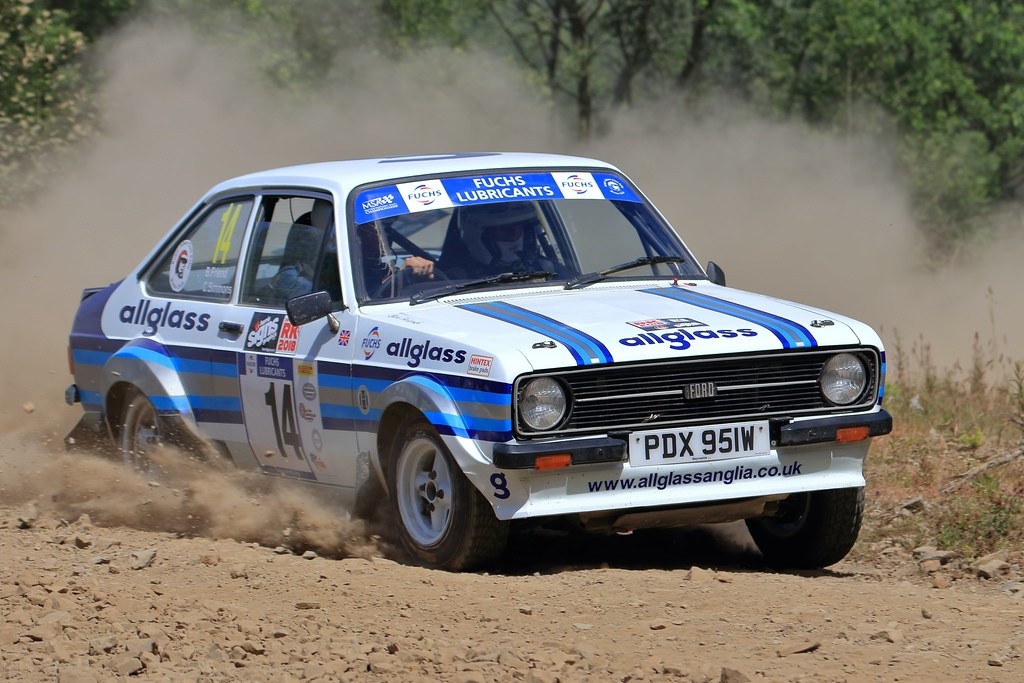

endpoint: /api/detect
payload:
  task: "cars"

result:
[63,150,894,576]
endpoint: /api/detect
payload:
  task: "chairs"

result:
[434,201,539,280]
[257,211,312,305]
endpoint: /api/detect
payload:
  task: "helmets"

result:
[310,198,398,258]
[458,201,539,265]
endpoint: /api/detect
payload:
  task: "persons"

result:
[266,211,437,313]
[469,204,563,285]
